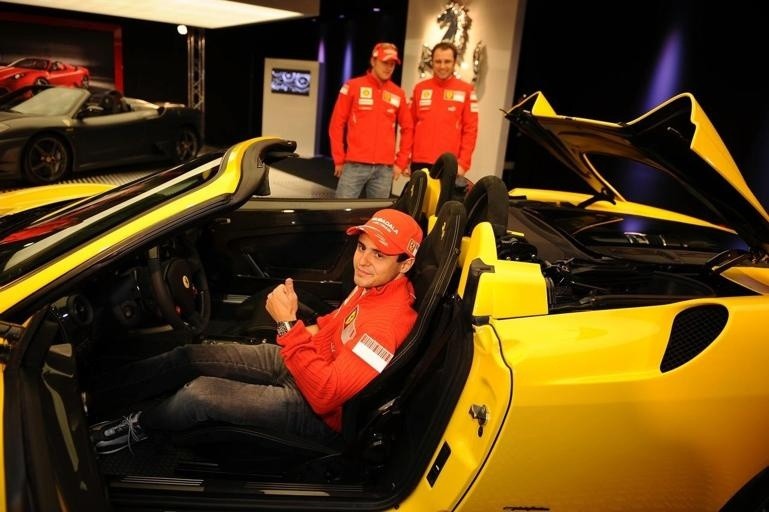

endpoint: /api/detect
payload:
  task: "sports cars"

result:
[0,85,212,184]
[0,89,769,512]
[0,56,91,102]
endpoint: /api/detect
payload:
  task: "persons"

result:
[87,208,423,455]
[402,42,479,184]
[328,43,413,200]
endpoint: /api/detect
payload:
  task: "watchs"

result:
[276,320,297,337]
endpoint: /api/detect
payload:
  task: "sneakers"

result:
[85,407,150,456]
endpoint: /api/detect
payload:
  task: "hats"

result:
[370,42,400,64]
[345,208,423,259]
